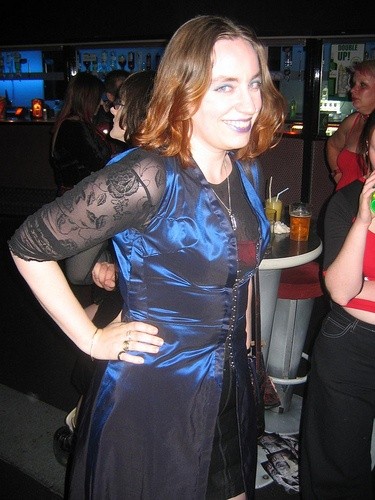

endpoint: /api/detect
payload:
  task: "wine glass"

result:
[283,47,293,67]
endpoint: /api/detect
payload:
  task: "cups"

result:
[263,207,276,255]
[289,201,313,242]
[338,72,351,93]
[265,198,282,220]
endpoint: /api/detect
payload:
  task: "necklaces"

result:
[210,160,238,232]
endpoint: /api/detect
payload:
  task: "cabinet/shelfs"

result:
[0,32,375,139]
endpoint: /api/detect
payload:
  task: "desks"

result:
[258,225,323,366]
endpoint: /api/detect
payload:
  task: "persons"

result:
[42,64,159,463]
[6,15,285,500]
[297,60,375,500]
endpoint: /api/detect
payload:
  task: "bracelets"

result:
[332,168,341,177]
[90,328,99,360]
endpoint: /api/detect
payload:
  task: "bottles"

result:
[0,51,23,74]
[43,107,48,120]
[289,96,296,119]
[83,51,152,73]
[328,57,337,97]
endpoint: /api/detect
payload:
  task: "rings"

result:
[122,341,129,351]
[118,351,125,360]
[126,331,131,340]
